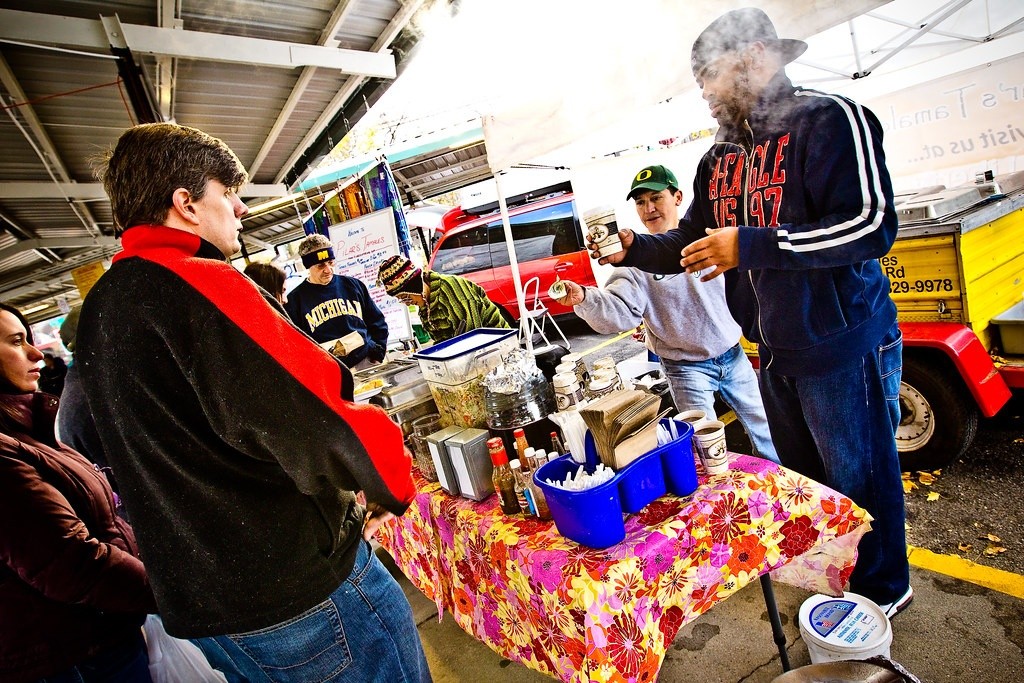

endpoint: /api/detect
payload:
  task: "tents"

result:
[478,0,1024,355]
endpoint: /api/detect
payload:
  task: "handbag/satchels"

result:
[140,613,229,683]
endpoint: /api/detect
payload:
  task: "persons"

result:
[378,255,520,345]
[584,7,915,621]
[553,164,780,466]
[0,123,431,683]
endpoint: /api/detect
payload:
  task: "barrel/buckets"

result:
[798,591,894,664]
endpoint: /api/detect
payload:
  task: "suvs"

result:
[424,192,599,338]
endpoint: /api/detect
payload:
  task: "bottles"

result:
[480,358,555,449]
[486,437,520,515]
[509,428,570,520]
[410,412,448,482]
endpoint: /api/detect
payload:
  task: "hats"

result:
[376,255,439,332]
[60,305,82,351]
[626,165,679,201]
[691,8,808,76]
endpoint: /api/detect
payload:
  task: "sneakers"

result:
[878,585,913,620]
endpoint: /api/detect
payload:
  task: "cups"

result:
[674,409,707,460]
[690,420,729,475]
[551,352,626,411]
[582,205,623,257]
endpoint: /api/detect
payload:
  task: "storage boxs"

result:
[532,415,697,549]
[413,325,520,429]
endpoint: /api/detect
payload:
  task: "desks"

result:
[372,448,875,683]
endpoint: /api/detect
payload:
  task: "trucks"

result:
[711,167,1024,471]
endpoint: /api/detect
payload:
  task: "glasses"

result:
[398,294,413,303]
